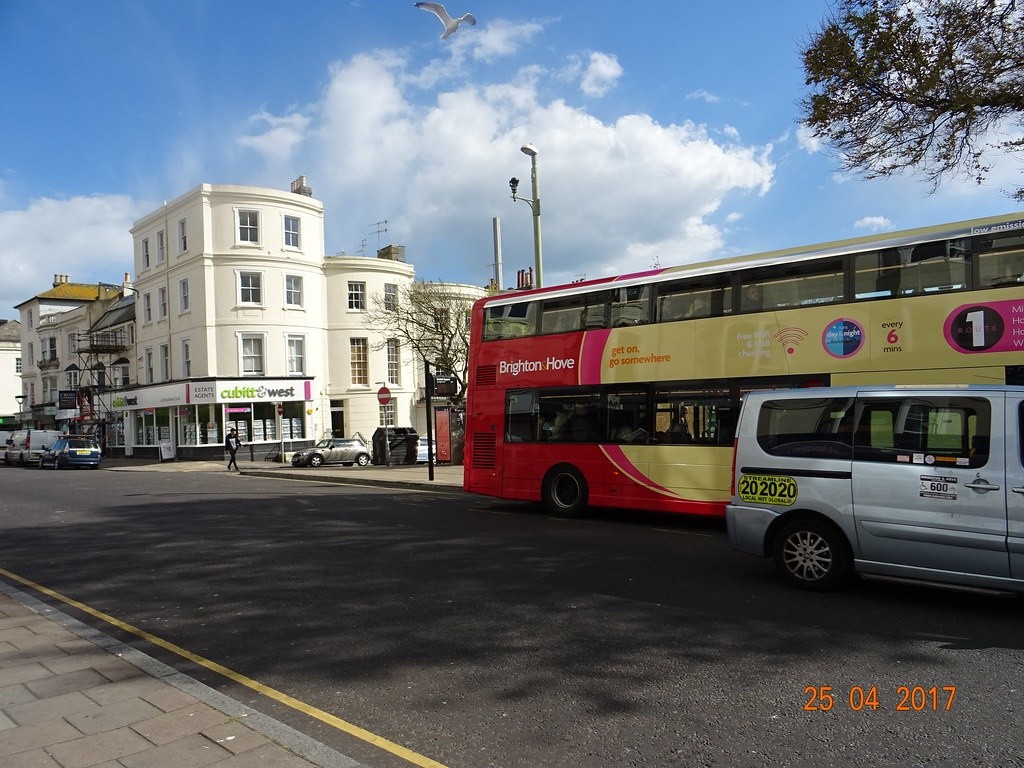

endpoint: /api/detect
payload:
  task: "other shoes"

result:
[227,465,231,470]
[235,468,240,471]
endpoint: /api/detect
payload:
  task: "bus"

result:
[462,213,1024,517]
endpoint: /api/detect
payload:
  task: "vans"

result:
[5,429,64,468]
[726,385,1024,595]
[0,430,13,462]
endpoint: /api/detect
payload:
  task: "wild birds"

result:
[413,2,477,40]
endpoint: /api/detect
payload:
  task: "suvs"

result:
[291,438,373,468]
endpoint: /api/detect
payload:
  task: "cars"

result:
[416,437,437,465]
[39,433,101,470]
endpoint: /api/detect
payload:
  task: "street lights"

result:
[507,143,543,291]
[374,381,392,466]
[14,395,25,430]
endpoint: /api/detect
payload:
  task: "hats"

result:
[230,428,236,431]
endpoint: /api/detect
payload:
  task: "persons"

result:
[742,284,763,311]
[486,302,606,338]
[909,279,927,293]
[533,401,693,443]
[225,428,243,471]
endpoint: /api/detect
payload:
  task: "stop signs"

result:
[377,386,391,404]
[277,404,283,416]
